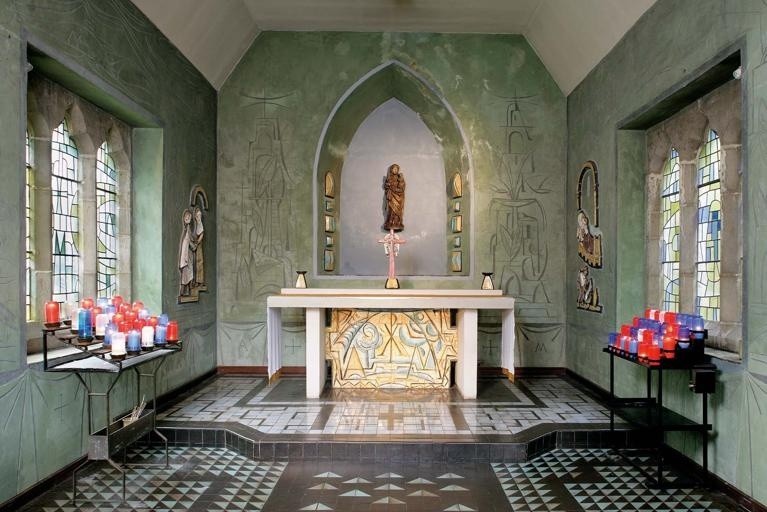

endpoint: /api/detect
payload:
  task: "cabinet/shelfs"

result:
[42,339,183,505]
[602,345,724,488]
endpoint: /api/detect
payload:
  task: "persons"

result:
[177,208,197,296]
[383,162,406,227]
[192,204,206,289]
[582,277,593,305]
[573,210,593,257]
[574,264,591,305]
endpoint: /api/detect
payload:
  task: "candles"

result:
[606,308,706,366]
[43,296,178,358]
